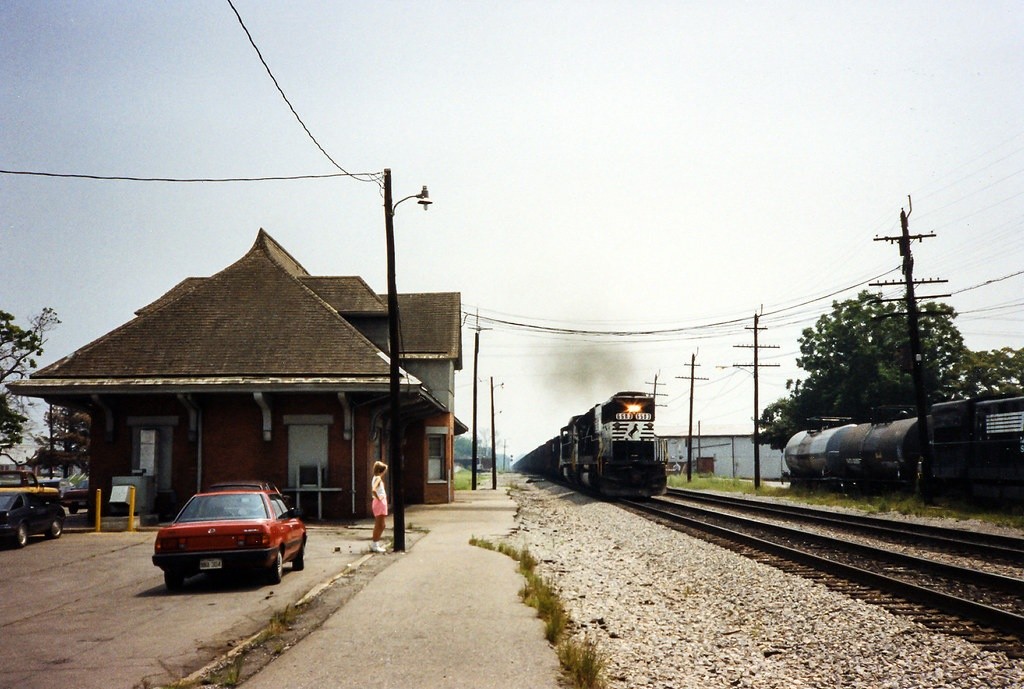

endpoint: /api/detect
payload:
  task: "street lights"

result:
[383,186,433,550]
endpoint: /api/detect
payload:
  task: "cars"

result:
[206,480,289,514]
[0,470,66,548]
[152,489,307,591]
[58,479,89,514]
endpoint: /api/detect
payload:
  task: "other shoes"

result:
[373,546,386,553]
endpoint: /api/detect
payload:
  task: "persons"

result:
[369,461,388,553]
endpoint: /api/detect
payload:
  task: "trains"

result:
[777,394,1024,512]
[512,391,668,501]
